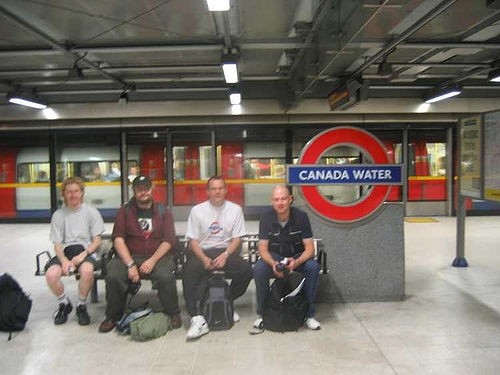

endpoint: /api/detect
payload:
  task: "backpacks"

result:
[201,273,235,331]
[0,272,32,341]
[263,271,311,333]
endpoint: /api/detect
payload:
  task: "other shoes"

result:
[171,314,183,328]
[75,304,90,325]
[54,297,73,324]
[99,315,117,333]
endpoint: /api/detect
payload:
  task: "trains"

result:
[0,136,500,218]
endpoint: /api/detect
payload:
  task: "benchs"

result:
[35,234,329,303]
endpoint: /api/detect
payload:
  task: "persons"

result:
[100,175,181,333]
[180,176,255,340]
[44,175,106,325]
[249,182,323,330]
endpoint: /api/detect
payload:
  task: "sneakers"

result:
[233,309,240,322]
[304,317,321,330]
[248,317,265,335]
[185,314,209,340]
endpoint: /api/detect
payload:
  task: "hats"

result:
[133,175,152,188]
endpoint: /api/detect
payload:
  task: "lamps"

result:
[118,86,129,105]
[222,47,241,85]
[228,86,242,105]
[64,51,88,81]
[6,88,48,110]
[377,46,398,76]
[424,80,464,104]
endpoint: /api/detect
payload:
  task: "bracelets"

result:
[127,262,136,269]
[86,250,89,254]
